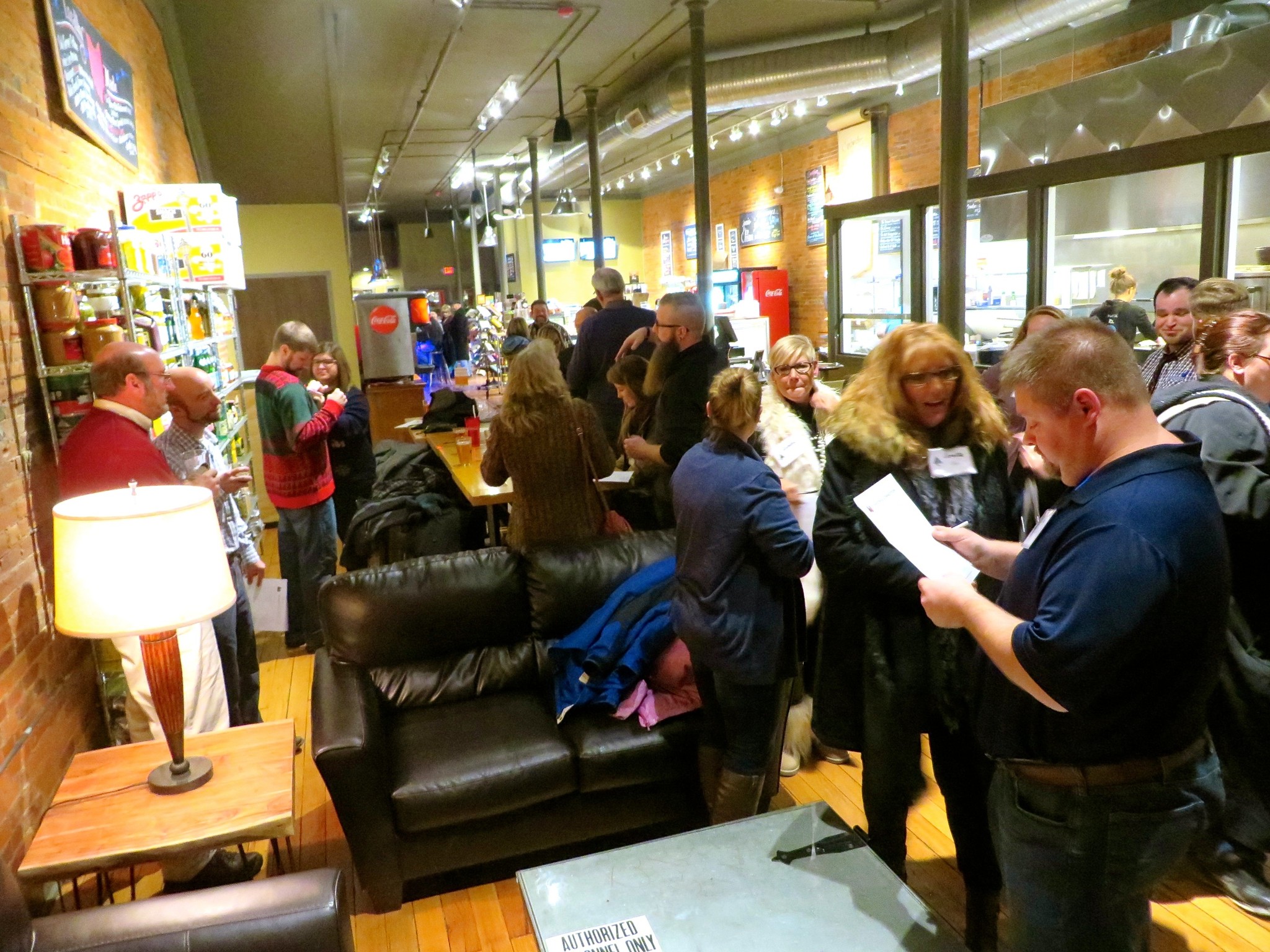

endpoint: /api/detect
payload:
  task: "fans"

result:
[493,154,546,220]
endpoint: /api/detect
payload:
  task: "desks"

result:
[405,416,637,547]
[18,719,297,914]
[515,800,971,952]
[818,362,844,382]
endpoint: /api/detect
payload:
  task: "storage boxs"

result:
[235,494,252,519]
[124,181,247,291]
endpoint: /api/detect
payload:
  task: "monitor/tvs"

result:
[578,236,617,260]
[543,238,575,263]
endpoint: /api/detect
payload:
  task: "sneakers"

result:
[164,849,263,892]
[1195,855,1270,917]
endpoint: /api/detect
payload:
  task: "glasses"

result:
[312,358,337,366]
[655,319,689,332]
[135,373,174,383]
[903,368,959,386]
[773,362,813,375]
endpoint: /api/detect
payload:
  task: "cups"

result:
[180,450,210,481]
[453,427,468,441]
[465,416,480,448]
[455,436,472,467]
[484,430,490,444]
[230,462,252,499]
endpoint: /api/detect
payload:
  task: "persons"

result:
[481,264,1270,952]
[254,316,377,656]
[59,339,268,739]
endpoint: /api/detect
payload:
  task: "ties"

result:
[1149,355,1175,393]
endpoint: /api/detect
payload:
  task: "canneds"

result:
[31,279,124,366]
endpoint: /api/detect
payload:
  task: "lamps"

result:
[478,117,488,131]
[551,57,572,142]
[478,181,498,247]
[422,200,434,239]
[53,478,239,794]
[709,94,828,150]
[549,147,586,216]
[600,145,693,193]
[363,150,390,222]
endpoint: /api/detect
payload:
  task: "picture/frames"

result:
[738,204,783,247]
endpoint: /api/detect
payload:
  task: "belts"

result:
[1009,740,1207,789]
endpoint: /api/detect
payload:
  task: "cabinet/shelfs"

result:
[8,208,267,747]
[966,264,1104,321]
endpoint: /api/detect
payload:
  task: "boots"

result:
[964,891,1002,952]
[779,695,814,776]
[816,743,849,764]
[699,743,766,826]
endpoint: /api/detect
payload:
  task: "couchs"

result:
[310,530,808,914]
[0,854,356,952]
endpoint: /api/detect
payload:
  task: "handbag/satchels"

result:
[603,510,634,537]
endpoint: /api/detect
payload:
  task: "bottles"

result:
[1011,291,1016,306]
[18,222,252,520]
[1000,291,1006,306]
